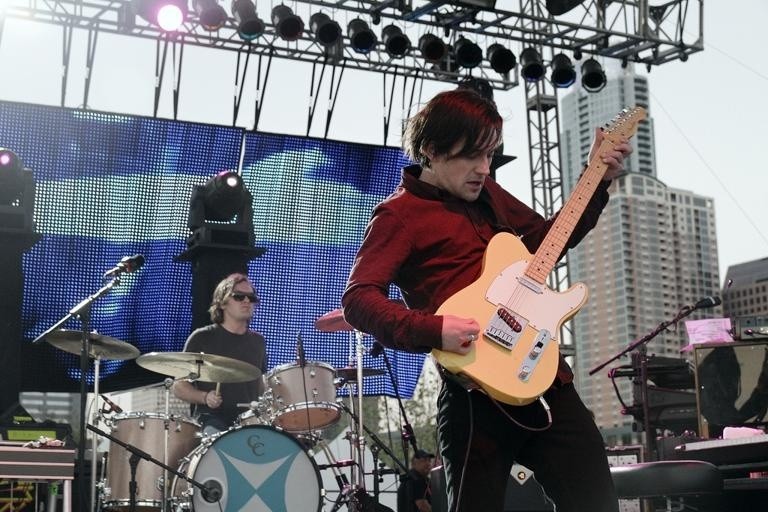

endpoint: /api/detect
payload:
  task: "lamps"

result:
[185,170,256,246]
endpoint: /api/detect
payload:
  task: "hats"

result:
[412,450,434,458]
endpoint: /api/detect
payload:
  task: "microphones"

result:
[695,296,722,309]
[100,394,121,416]
[104,254,145,278]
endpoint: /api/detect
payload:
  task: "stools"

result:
[610,459,724,512]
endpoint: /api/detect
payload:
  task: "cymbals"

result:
[333,366,388,384]
[45,328,141,361]
[314,298,407,332]
[136,351,262,384]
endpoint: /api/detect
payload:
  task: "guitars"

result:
[433,104,647,406]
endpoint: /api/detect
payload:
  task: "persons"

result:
[393,449,440,510]
[173,273,266,437]
[339,89,632,512]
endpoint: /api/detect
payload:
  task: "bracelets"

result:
[202,392,208,406]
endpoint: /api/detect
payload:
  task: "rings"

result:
[468,334,474,341]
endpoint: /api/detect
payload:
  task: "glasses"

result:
[231,292,261,302]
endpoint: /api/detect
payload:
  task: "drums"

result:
[262,359,341,432]
[99,411,202,512]
[169,421,325,512]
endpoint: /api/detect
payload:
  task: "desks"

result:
[0,446,76,512]
